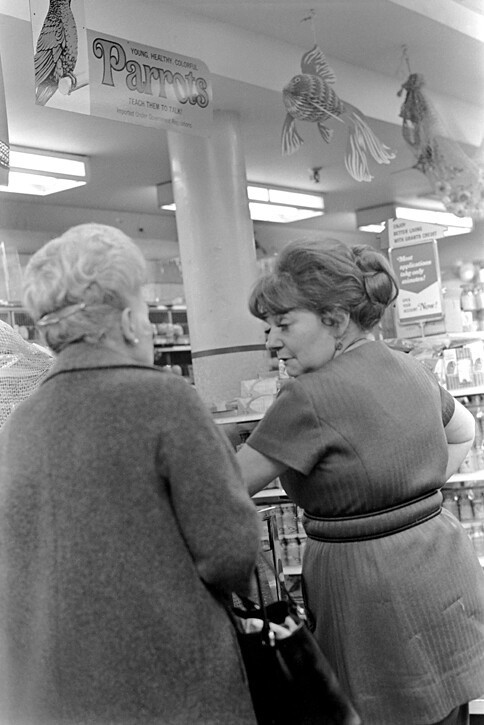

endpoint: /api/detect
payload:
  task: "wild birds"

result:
[33,0,90,107]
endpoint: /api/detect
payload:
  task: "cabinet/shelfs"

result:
[207,385,484,715]
[0,299,196,388]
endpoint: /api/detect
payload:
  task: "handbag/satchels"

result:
[229,550,361,725]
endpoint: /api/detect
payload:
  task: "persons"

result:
[0,223,260,725]
[234,236,484,725]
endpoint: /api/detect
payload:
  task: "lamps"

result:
[157,181,327,223]
[355,203,473,237]
[0,145,90,196]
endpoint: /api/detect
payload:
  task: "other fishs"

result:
[280,44,395,182]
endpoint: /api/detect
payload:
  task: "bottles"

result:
[272,506,308,569]
[457,396,484,473]
[460,284,484,332]
[442,490,484,559]
